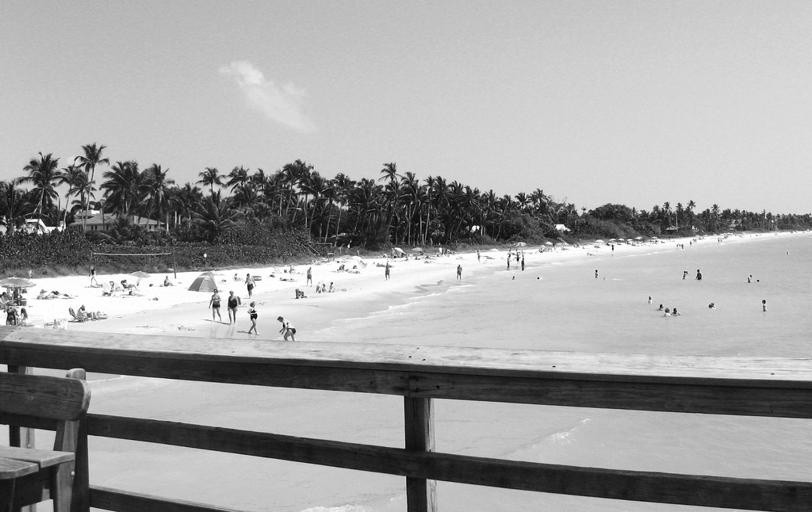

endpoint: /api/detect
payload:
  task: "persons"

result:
[336,264,361,274]
[647,268,768,318]
[595,269,598,278]
[287,264,334,299]
[209,288,239,325]
[247,301,259,335]
[456,265,463,280]
[244,273,257,298]
[277,316,296,341]
[384,263,391,280]
[1,265,172,326]
[506,247,525,271]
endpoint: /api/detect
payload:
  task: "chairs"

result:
[0,364,92,511]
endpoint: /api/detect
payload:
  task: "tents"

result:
[188,271,218,292]
